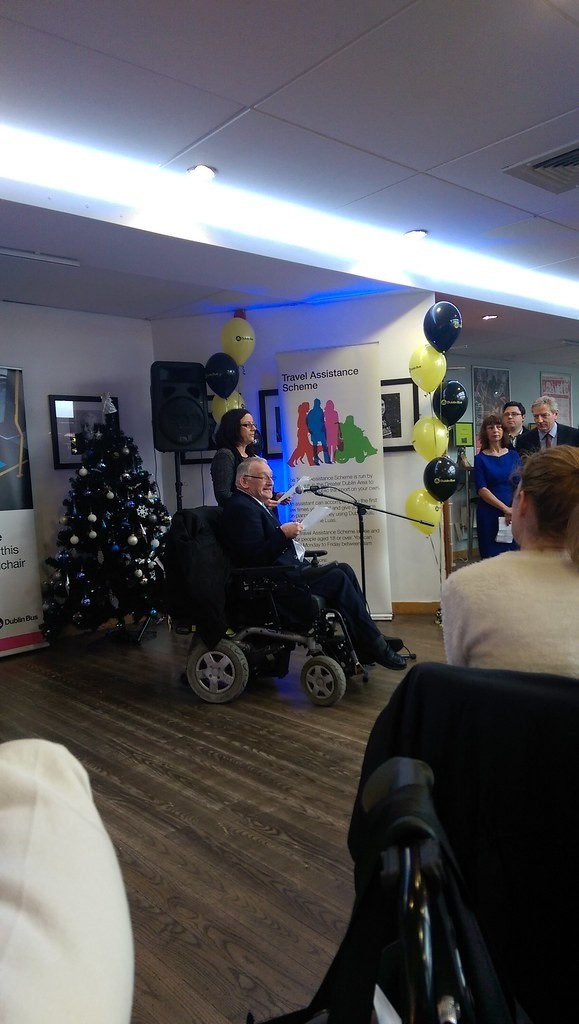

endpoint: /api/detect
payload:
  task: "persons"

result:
[210,409,294,517]
[516,395,579,456]
[473,414,522,559]
[441,445,579,677]
[219,457,407,670]
[503,401,528,449]
[381,398,393,438]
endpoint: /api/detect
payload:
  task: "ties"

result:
[545,433,551,448]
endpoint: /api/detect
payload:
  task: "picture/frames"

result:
[540,371,573,427]
[179,393,242,465]
[258,389,284,460]
[381,378,420,453]
[470,366,511,459]
[48,394,118,469]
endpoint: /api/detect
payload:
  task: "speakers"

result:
[151,361,210,453]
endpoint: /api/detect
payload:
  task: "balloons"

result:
[409,346,447,393]
[221,318,255,366]
[205,352,239,400]
[423,457,459,502]
[211,391,245,425]
[432,381,468,427]
[411,417,449,461]
[423,302,462,355]
[405,489,443,536]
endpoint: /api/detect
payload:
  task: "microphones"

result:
[295,485,332,495]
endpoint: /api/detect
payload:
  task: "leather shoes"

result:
[375,646,407,670]
[384,636,403,651]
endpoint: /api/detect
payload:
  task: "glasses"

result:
[503,412,522,417]
[244,475,274,481]
[239,424,257,429]
